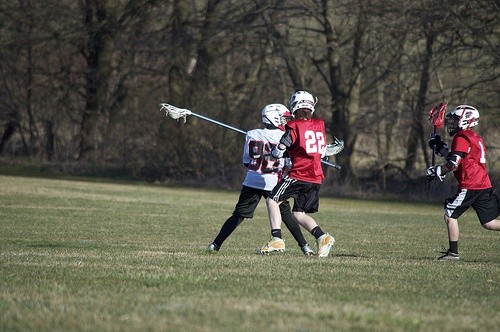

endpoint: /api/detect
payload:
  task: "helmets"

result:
[289,91,315,115]
[446,105,480,137]
[262,104,290,131]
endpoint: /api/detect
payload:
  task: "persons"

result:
[426,105,500,259]
[258,90,335,257]
[209,103,317,255]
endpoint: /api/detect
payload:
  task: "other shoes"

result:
[207,244,217,254]
[301,244,316,256]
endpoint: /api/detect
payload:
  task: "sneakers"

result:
[259,236,285,254]
[316,232,335,257]
[436,249,459,261]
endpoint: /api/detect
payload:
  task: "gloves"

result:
[428,133,449,157]
[426,165,446,182]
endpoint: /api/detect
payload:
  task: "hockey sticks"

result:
[159,102,341,170]
[324,140,344,157]
[429,102,446,181]
